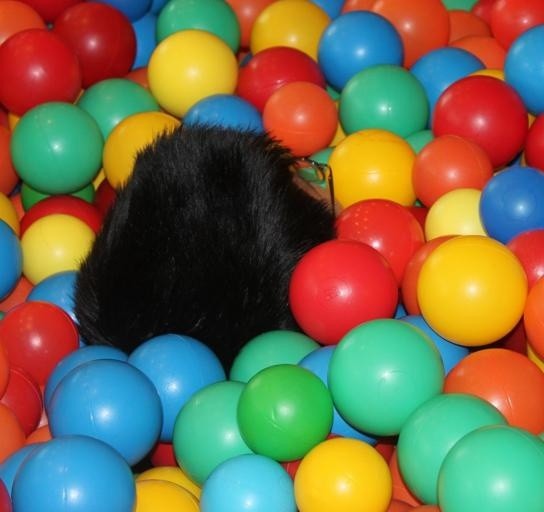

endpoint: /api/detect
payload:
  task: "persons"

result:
[70,121,339,380]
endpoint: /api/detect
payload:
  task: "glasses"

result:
[293,155,335,221]
